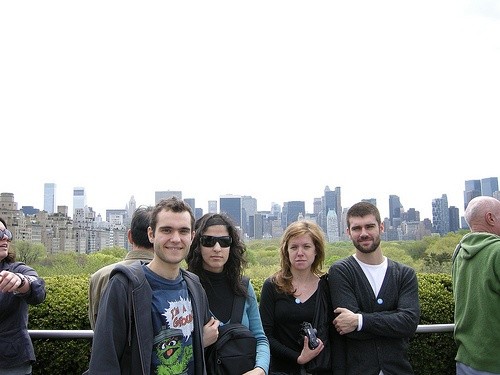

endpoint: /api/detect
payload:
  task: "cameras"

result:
[296,321,318,349]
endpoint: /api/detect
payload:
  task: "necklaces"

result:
[294,279,318,304]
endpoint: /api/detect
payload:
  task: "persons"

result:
[0,217,47,375]
[327,201,420,375]
[259,220,346,375]
[82,196,214,375]
[88,205,155,333]
[451,196,500,375]
[185,213,270,375]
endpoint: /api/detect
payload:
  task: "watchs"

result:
[15,272,25,288]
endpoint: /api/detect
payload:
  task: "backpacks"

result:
[206,276,257,375]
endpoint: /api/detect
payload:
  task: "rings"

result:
[0,274,5,278]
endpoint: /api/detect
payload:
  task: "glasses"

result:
[0,229,13,242]
[199,235,233,248]
